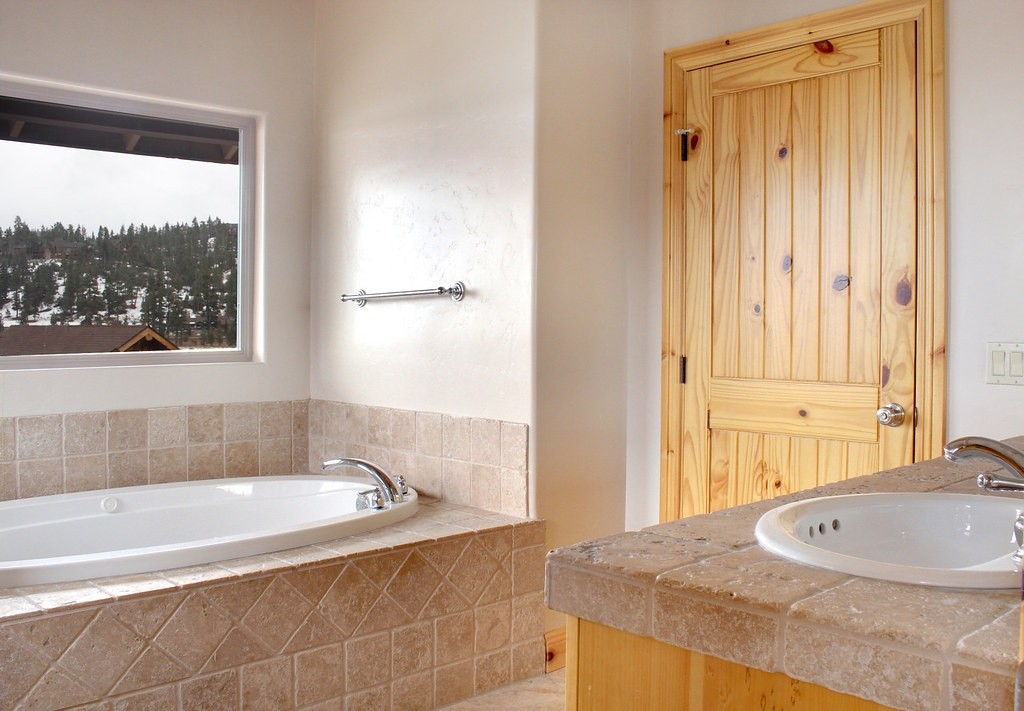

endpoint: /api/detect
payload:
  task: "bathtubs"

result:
[1,474,419,590]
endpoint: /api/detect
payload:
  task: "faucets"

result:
[945,436,1024,481]
[322,458,402,507]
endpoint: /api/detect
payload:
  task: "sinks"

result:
[756,489,1021,590]
